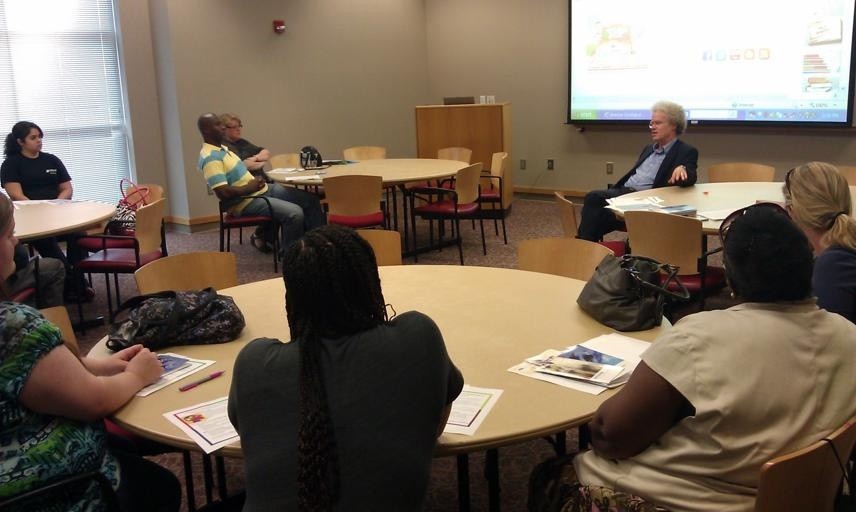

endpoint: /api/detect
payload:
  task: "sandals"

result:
[249,233,273,254]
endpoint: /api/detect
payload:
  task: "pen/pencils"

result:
[178,369,226,392]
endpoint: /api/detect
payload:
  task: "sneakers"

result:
[63,286,95,301]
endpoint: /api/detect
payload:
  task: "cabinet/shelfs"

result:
[414,102,514,220]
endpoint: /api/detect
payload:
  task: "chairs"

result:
[344,146,386,160]
[437,147,472,165]
[709,162,775,182]
[75,197,167,336]
[41,307,215,512]
[79,184,163,253]
[624,211,728,311]
[411,162,487,265]
[270,153,302,169]
[134,251,238,300]
[219,196,278,273]
[451,152,512,244]
[517,238,614,282]
[6,255,42,309]
[755,416,855,512]
[0,470,118,512]
[355,230,401,266]
[320,175,387,229]
[555,191,626,257]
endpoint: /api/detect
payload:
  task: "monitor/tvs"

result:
[444,96,475,104]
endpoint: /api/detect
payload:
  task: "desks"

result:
[266,158,470,187]
[612,182,785,265]
[84,265,675,512]
[13,199,117,239]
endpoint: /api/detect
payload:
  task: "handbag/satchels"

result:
[300,145,323,169]
[575,252,694,332]
[106,286,247,353]
[108,178,150,237]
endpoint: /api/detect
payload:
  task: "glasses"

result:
[785,165,805,197]
[717,202,795,274]
[227,125,243,131]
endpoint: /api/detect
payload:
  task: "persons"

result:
[527,203,856,512]
[1,194,181,511]
[0,121,95,304]
[197,112,305,255]
[782,163,856,320]
[227,226,463,511]
[219,114,326,253]
[574,102,697,243]
